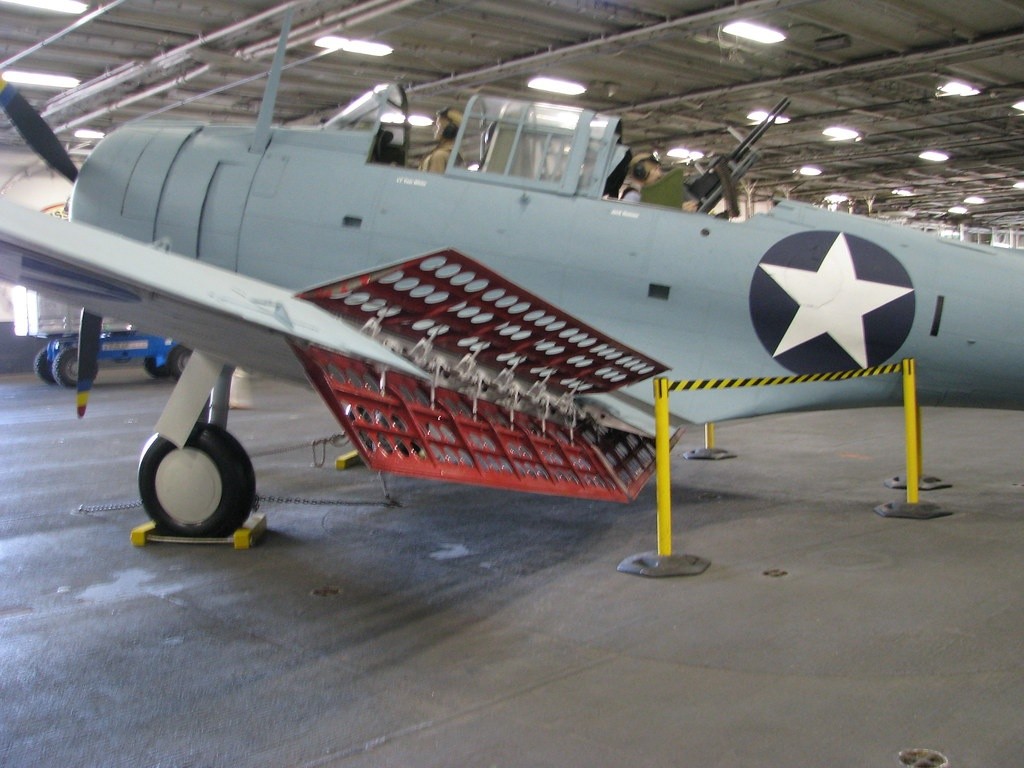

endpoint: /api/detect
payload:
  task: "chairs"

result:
[475,116,498,171]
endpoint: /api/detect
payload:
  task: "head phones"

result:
[439,108,459,139]
[634,157,660,180]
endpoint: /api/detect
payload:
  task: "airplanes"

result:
[0,0,1024,538]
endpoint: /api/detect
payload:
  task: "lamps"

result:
[0,0,1024,219]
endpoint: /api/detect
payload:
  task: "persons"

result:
[392,106,467,174]
[616,152,700,213]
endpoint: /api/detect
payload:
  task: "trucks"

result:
[12,261,193,388]
